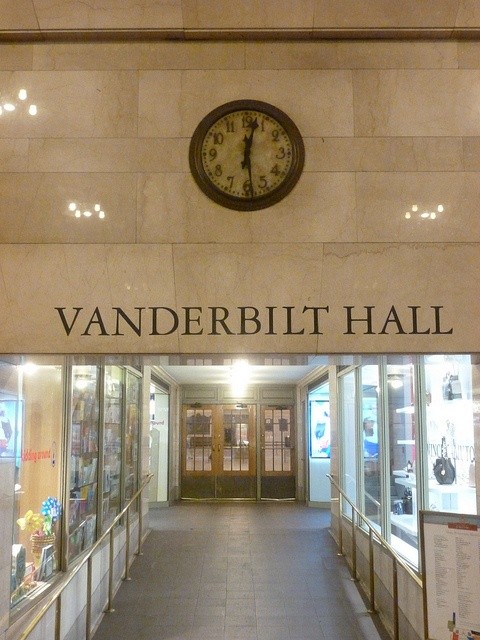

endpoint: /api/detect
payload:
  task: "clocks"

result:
[187,98,305,213]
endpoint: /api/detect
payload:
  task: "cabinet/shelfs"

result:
[391,363,475,536]
[68,366,143,564]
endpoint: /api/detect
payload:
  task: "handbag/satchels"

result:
[443,372,462,401]
[433,437,455,484]
